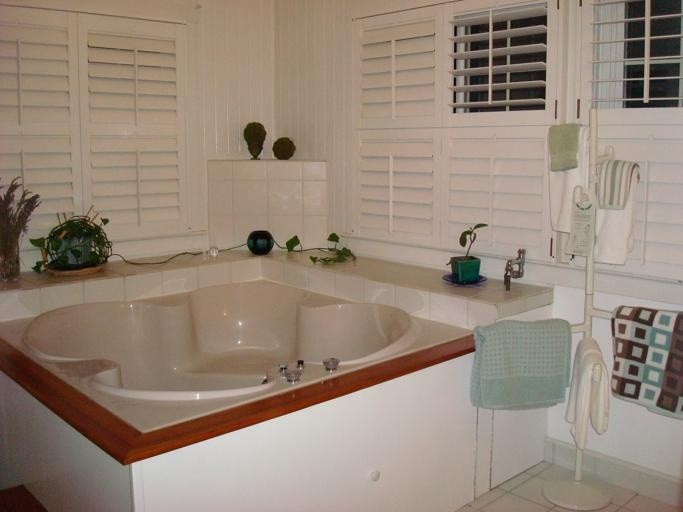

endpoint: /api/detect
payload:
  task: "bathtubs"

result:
[23,281,418,400]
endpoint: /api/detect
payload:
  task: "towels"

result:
[548,123,580,172]
[565,338,611,452]
[471,318,569,409]
[609,306,682,422]
[593,158,641,269]
[548,173,580,266]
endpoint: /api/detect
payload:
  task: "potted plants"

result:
[0,177,39,282]
[444,220,487,283]
